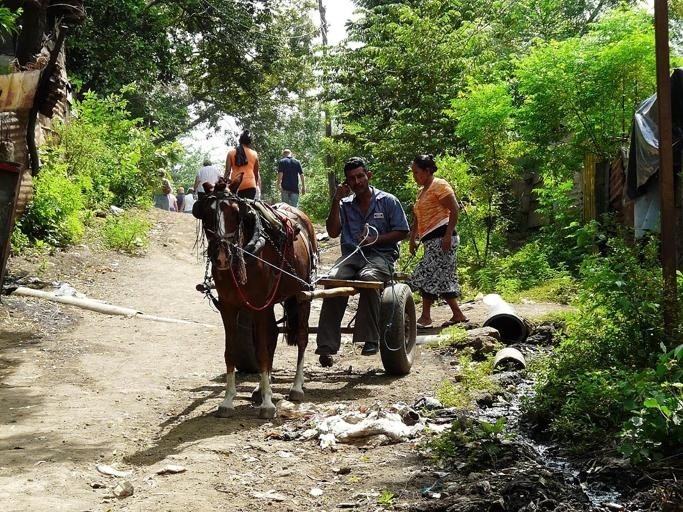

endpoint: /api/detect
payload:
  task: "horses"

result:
[198,172,321,419]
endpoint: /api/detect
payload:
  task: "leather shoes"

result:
[362,342,378,355]
[315,345,337,355]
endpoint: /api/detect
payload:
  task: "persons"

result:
[315,157,410,367]
[152,131,305,212]
[409,155,469,328]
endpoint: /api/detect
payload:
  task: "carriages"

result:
[191,172,416,419]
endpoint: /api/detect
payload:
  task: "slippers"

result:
[417,318,469,329]
